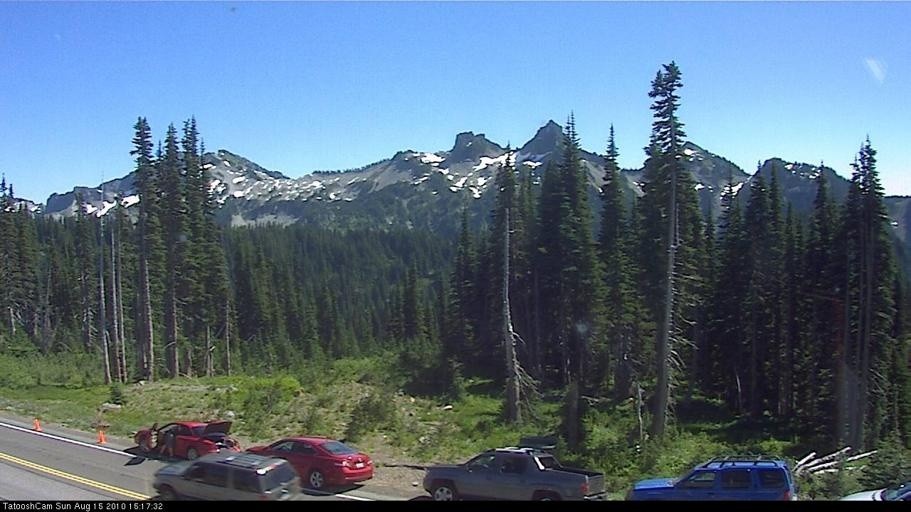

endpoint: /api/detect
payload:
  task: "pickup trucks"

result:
[423,447,608,501]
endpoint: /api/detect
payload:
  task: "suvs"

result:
[624,455,798,501]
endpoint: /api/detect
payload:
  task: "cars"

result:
[133,420,374,502]
